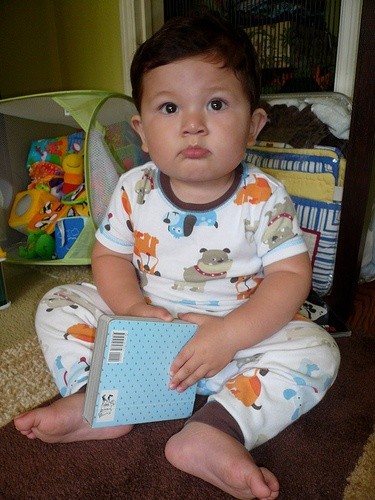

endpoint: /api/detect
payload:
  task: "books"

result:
[81,314,199,429]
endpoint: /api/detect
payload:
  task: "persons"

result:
[13,18,340,500]
